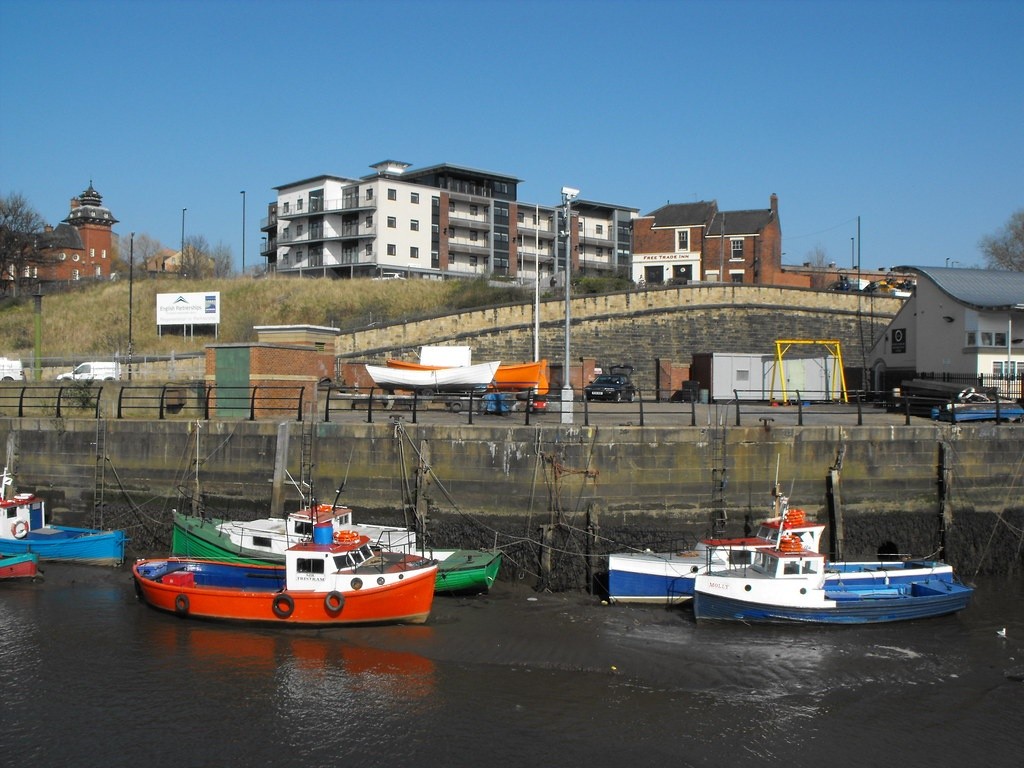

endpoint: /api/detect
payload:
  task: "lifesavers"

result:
[325,591,345,612]
[958,387,976,399]
[135,579,143,599]
[784,509,806,526]
[316,504,332,512]
[11,518,29,538]
[176,594,188,611]
[333,529,360,545]
[779,535,803,552]
[273,594,294,617]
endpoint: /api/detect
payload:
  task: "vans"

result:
[0,360,26,381]
[56,362,122,380]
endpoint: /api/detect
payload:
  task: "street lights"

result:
[240,190,246,275]
[260,236,266,271]
[181,207,186,272]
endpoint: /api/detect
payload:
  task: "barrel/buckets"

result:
[533,394,546,415]
[699,389,708,404]
[314,523,333,545]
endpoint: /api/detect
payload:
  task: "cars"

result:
[585,365,637,403]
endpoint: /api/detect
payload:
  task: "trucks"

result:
[827,277,915,296]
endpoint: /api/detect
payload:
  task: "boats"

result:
[382,358,550,397]
[0,550,40,578]
[606,452,952,605]
[131,496,438,625]
[364,360,500,394]
[693,519,975,622]
[165,501,501,594]
[0,468,125,565]
